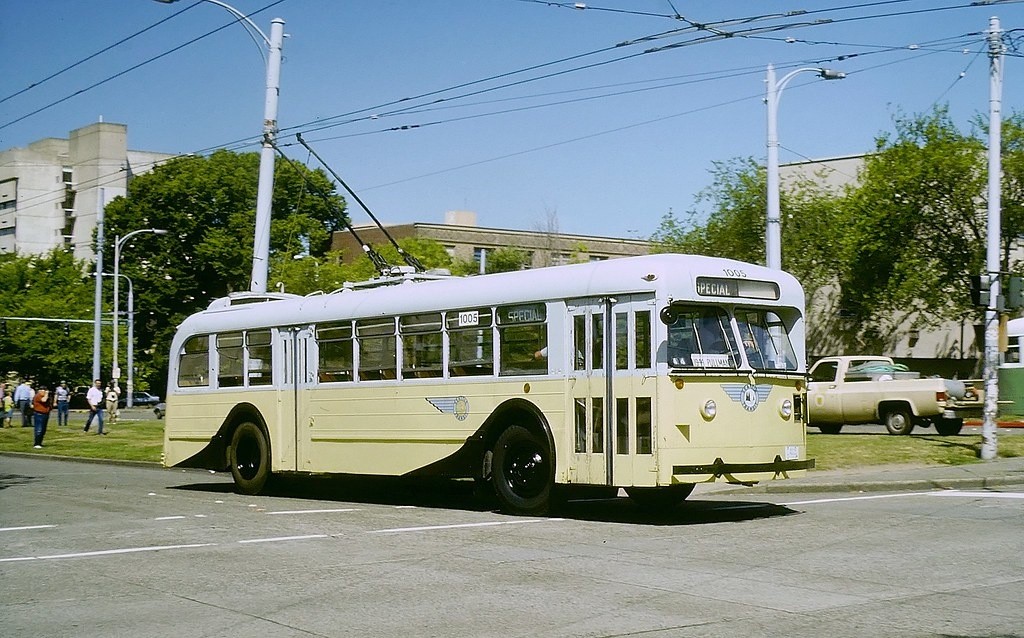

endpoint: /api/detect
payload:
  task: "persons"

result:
[83,379,105,435]
[1,391,16,426]
[535,346,585,452]
[32,386,52,448]
[0,381,8,427]
[53,380,72,426]
[104,380,121,423]
[14,380,35,428]
[699,311,754,354]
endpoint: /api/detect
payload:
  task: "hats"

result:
[39,386,47,390]
[61,380,66,384]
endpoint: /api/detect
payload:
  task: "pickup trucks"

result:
[804,355,986,435]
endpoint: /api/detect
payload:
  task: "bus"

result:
[160,132,815,517]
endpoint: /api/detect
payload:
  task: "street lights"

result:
[765,61,846,368]
[112,228,169,388]
[93,272,134,408]
[156,0,286,293]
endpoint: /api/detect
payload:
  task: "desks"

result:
[507,358,544,369]
[317,367,353,382]
[450,359,493,375]
[401,366,444,379]
[358,365,397,379]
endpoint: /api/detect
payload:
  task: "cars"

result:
[153,399,166,420]
[47,386,92,410]
[117,392,160,409]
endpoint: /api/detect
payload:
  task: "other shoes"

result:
[21,423,33,427]
[98,432,106,435]
[84,428,87,434]
[34,445,42,449]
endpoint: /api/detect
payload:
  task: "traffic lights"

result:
[969,273,991,308]
